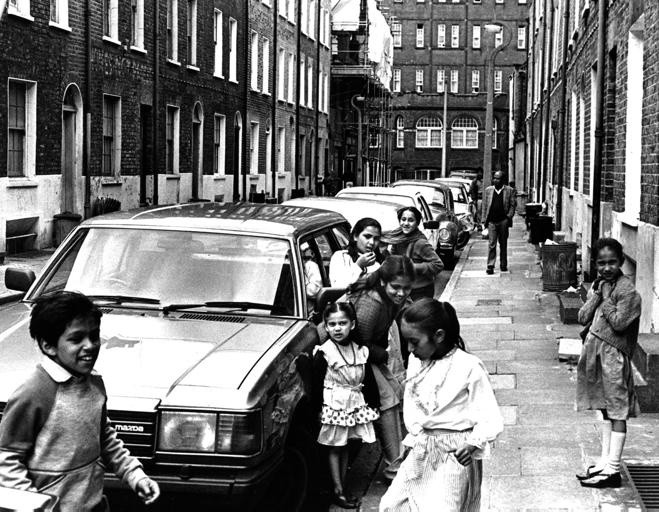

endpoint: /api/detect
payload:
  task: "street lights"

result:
[351,94,365,185]
[480,22,514,228]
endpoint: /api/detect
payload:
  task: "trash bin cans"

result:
[54,211,82,248]
[542,242,578,291]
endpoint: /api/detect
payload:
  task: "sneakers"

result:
[332,489,358,508]
[501,267,507,271]
[486,269,494,274]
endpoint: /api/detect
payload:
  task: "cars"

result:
[387,178,462,271]
[280,195,407,232]
[335,186,441,254]
[432,168,477,250]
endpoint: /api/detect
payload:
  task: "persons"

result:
[0,291,162,512]
[376,296,503,512]
[300,205,444,509]
[572,236,642,488]
[480,169,517,274]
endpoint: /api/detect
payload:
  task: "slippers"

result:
[580,472,622,488]
[576,466,604,480]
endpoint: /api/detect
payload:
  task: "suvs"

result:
[0,201,352,512]
